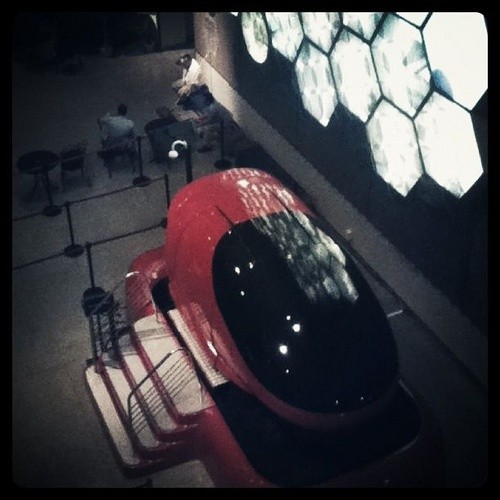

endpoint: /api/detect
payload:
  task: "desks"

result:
[18,150,59,202]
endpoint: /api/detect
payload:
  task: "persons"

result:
[171,54,202,106]
[192,90,224,153]
[161,97,203,134]
[100,103,135,146]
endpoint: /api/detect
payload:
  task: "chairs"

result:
[98,131,138,176]
[148,122,191,167]
[60,146,90,193]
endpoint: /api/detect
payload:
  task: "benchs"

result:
[191,87,254,155]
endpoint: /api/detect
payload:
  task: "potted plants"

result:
[98,13,115,57]
[142,12,161,52]
